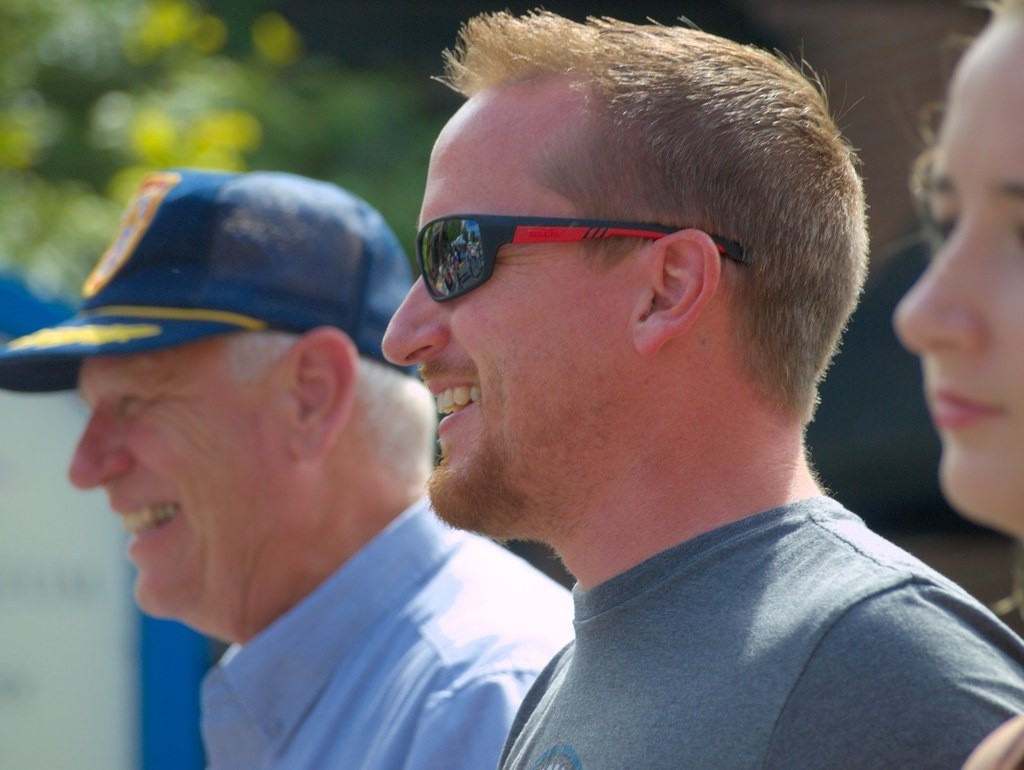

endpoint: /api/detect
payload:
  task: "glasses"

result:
[416,215,757,302]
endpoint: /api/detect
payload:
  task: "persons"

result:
[383,10,1024,770]
[1,170,574,770]
[892,0,1024,770]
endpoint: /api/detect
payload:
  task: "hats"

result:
[0,170,421,395]
